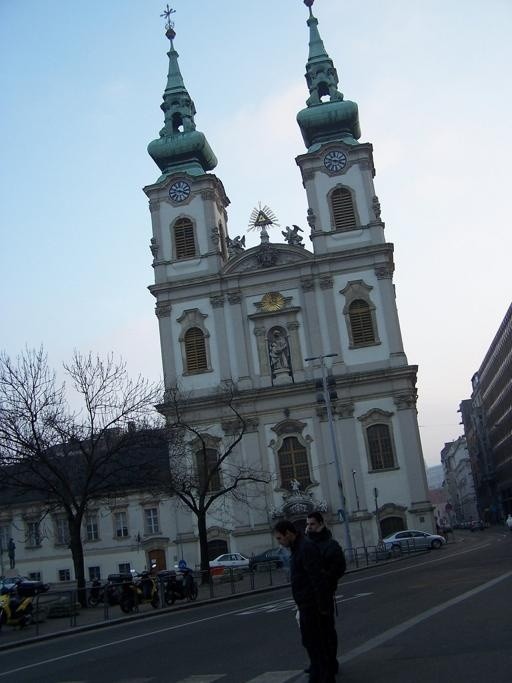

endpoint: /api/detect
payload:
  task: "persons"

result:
[506,513,512,530]
[292,513,346,671]
[270,343,287,370]
[7,536,18,570]
[272,330,288,369]
[271,518,338,682]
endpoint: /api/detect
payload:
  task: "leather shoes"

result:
[304,665,338,674]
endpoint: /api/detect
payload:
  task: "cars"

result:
[0,575,44,597]
[249,546,292,571]
[376,528,449,557]
[209,552,253,570]
[438,520,485,534]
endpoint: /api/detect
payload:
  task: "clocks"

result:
[166,181,193,204]
[324,151,349,173]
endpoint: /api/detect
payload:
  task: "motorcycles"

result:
[88,561,200,613]
[0,578,38,626]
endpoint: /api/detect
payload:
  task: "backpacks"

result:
[304,537,346,580]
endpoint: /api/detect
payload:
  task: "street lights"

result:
[304,352,356,565]
